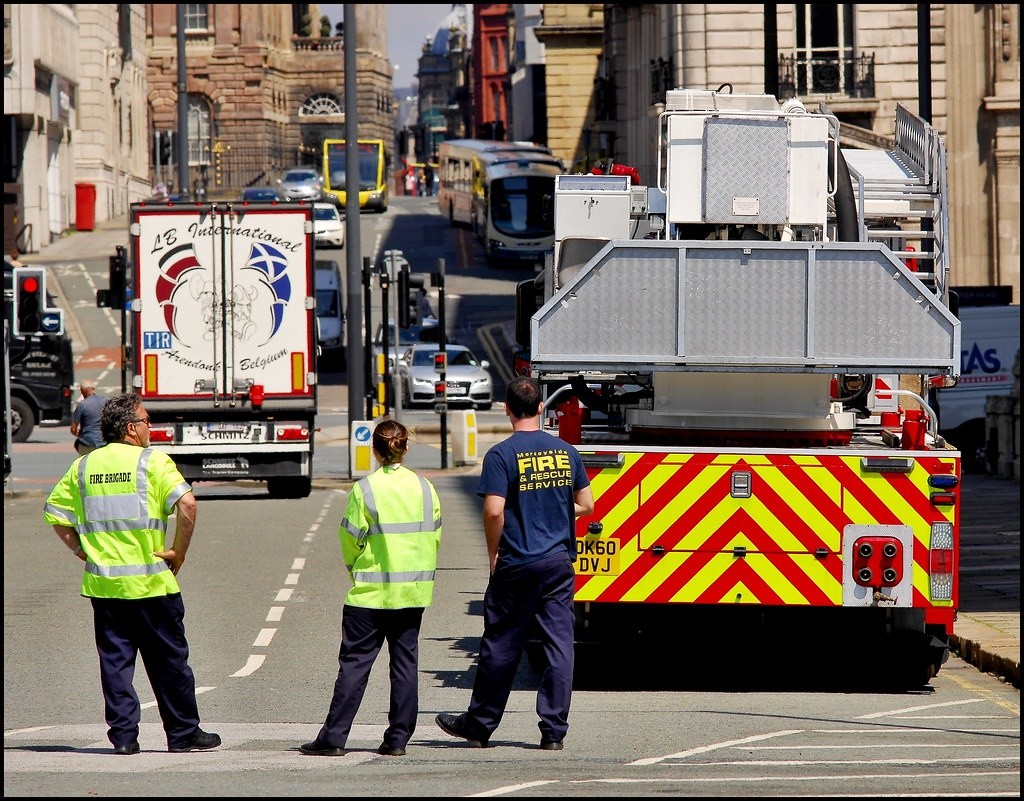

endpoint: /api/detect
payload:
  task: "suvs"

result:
[2,257,76,445]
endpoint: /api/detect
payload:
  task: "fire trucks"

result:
[476,81,966,683]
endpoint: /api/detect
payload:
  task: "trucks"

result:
[121,197,318,500]
[935,303,1019,476]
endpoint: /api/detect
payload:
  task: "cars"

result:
[311,203,346,250]
[368,318,459,394]
[275,168,324,202]
[391,342,494,413]
[241,187,280,201]
[314,258,347,370]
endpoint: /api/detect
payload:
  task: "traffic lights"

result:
[152,132,171,165]
[97,242,128,311]
[11,265,64,337]
[397,264,425,329]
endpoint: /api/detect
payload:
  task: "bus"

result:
[321,138,392,213]
[435,139,567,272]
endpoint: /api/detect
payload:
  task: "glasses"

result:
[125,415,150,430]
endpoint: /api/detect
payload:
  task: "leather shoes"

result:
[168,727,222,752]
[436,713,489,747]
[378,741,406,755]
[114,739,139,754]
[539,737,564,750]
[299,739,345,755]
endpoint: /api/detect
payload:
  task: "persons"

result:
[402,161,433,198]
[436,378,593,750]
[71,378,112,456]
[299,420,442,756]
[42,394,222,755]
